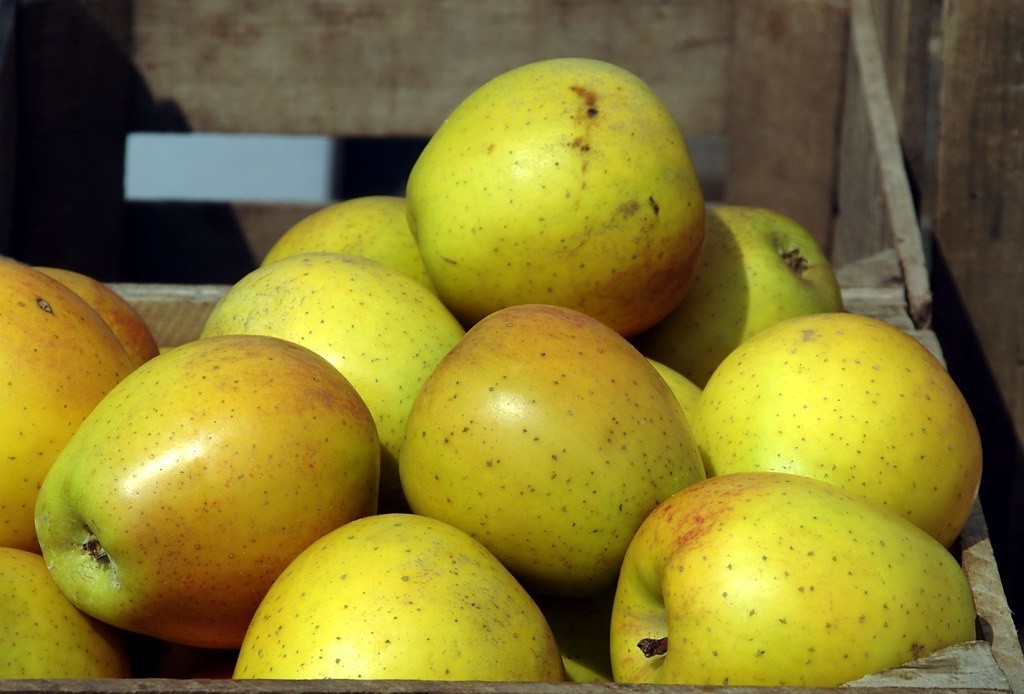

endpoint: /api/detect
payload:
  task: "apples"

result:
[0,55,983,694]
